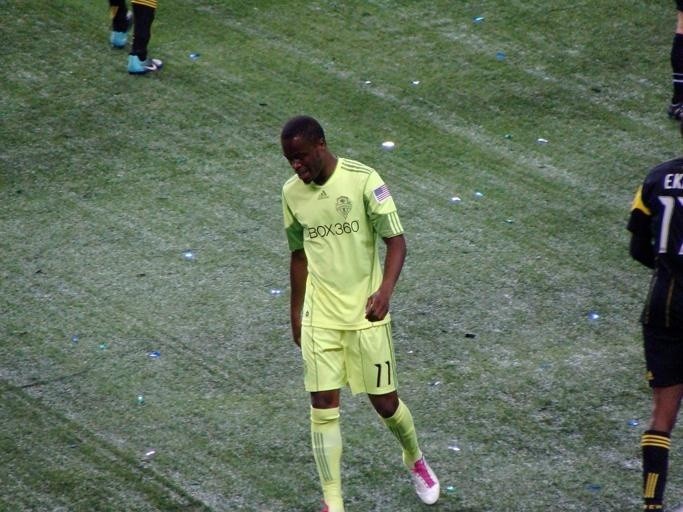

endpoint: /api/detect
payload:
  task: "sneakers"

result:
[402,450,441,505]
[668,103,683,120]
[109,10,134,49]
[127,54,163,74]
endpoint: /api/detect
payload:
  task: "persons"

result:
[668,0,682,121]
[107,1,163,77]
[278,116,444,512]
[626,155,682,511]
